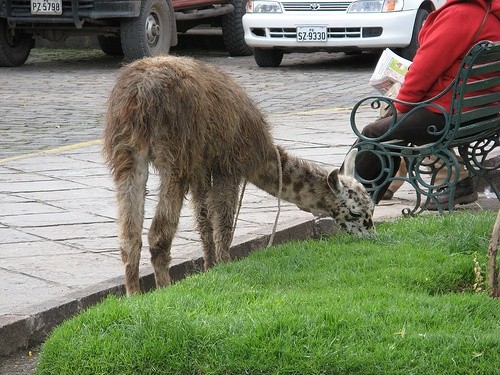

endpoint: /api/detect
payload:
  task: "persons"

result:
[338,0,500,206]
[380,148,478,211]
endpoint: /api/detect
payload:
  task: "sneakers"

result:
[419,176,478,210]
[382,189,393,200]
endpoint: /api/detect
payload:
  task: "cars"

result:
[0,0,254,67]
[242,0,446,67]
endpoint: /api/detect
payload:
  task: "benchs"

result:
[344,39,500,218]
